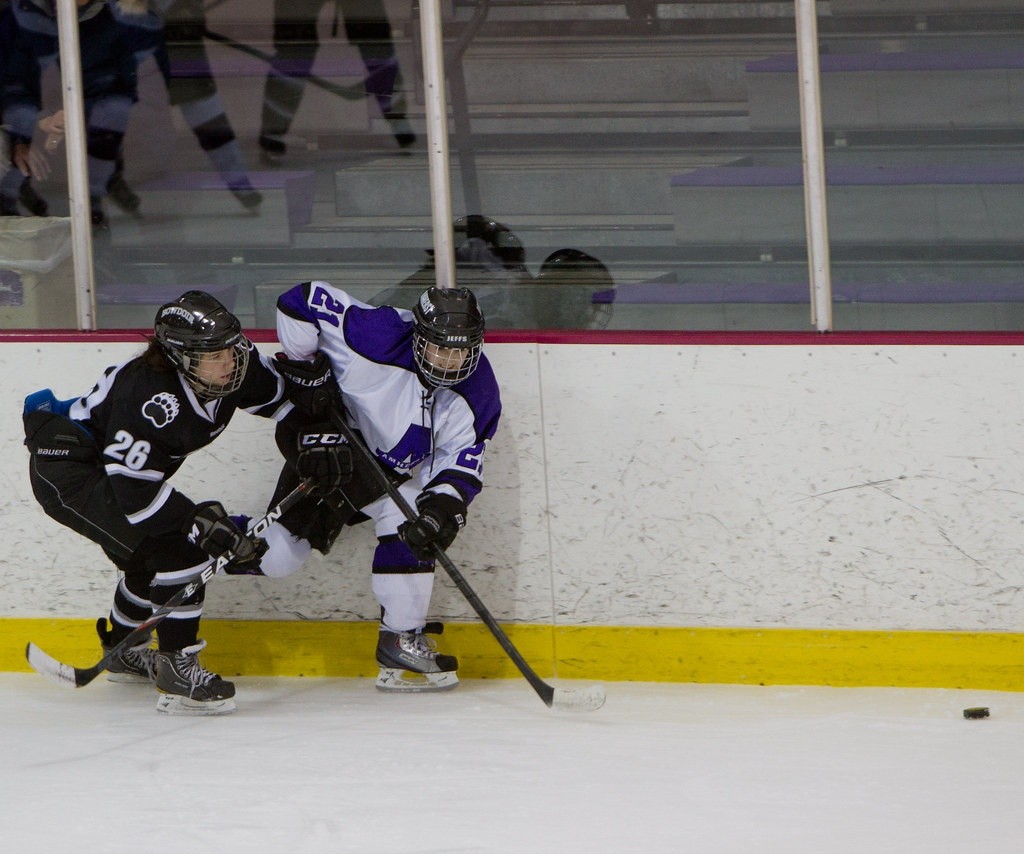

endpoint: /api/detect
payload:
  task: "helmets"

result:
[436,214,526,277]
[411,287,484,389]
[154,290,249,402]
[541,248,616,331]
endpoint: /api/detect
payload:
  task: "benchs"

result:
[589,0,1024,330]
[1,0,456,328]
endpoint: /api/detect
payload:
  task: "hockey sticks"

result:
[24,475,313,689]
[321,396,606,712]
[193,22,400,100]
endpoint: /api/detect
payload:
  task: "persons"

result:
[0,0,263,230]
[22,289,353,716]
[258,0,417,169]
[365,214,617,328]
[208,280,502,692]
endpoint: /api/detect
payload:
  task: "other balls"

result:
[964,707,991,719]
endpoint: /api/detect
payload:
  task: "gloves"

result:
[397,504,459,562]
[297,428,354,500]
[182,501,269,566]
[271,349,343,422]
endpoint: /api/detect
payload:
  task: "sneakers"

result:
[155,640,236,715]
[229,185,265,216]
[375,621,459,693]
[106,176,143,220]
[96,618,159,684]
